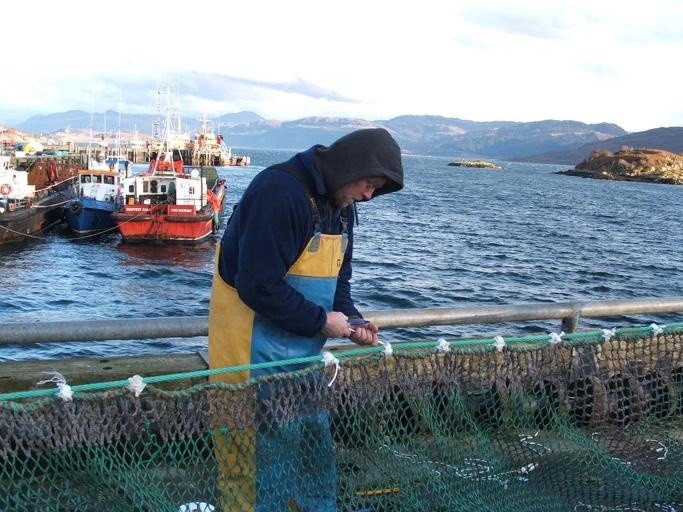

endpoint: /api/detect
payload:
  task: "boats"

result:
[111,82,228,247]
[0,151,80,248]
[61,105,134,236]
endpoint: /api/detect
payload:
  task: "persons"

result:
[208,127,404,512]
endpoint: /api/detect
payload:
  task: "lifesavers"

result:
[1,184,12,195]
